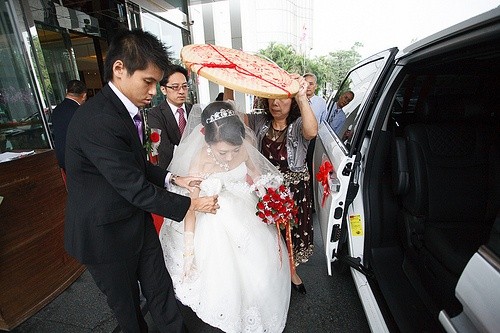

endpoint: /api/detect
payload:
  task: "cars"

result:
[5,105,57,148]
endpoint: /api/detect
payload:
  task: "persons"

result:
[326,90,354,136]
[51,28,326,333]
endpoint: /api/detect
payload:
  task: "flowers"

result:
[256,178,299,227]
[143,128,162,160]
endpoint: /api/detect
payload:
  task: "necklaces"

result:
[272,121,287,141]
[211,151,230,171]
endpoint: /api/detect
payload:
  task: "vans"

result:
[310,5,500,333]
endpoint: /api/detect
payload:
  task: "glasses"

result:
[164,84,188,91]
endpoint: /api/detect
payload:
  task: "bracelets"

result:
[183,253,194,256]
[171,174,180,186]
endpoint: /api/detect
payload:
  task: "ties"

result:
[177,108,185,134]
[133,112,143,145]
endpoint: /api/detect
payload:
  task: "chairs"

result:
[391,81,500,318]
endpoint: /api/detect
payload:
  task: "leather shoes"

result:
[292,272,307,296]
